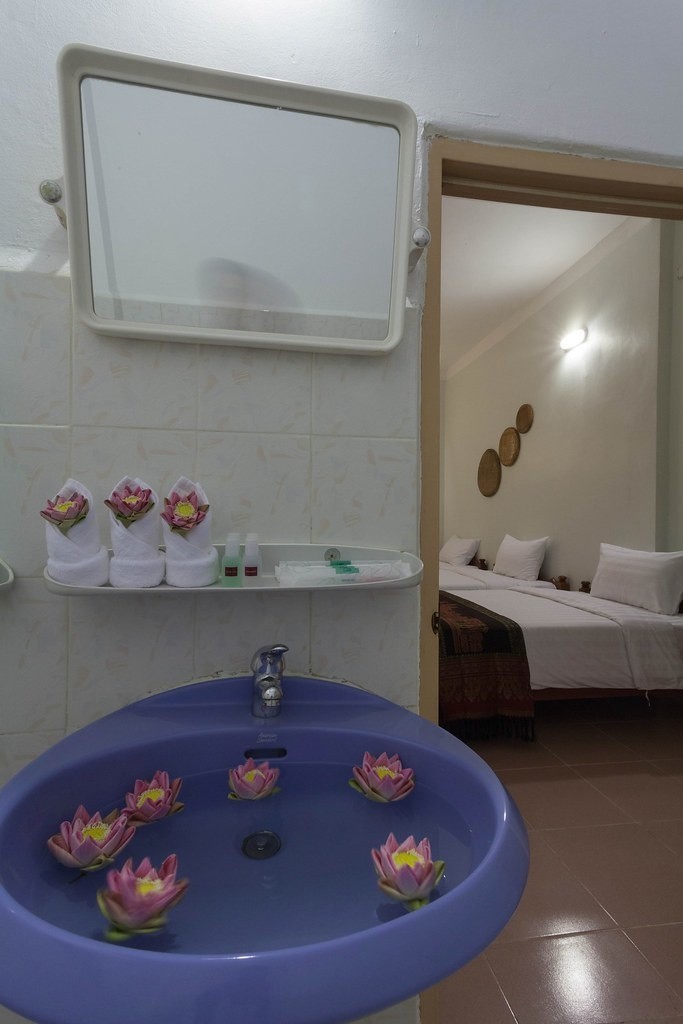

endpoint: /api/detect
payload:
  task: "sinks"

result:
[1,672,529,1024]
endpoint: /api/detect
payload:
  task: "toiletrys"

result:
[221,532,243,588]
[243,530,263,587]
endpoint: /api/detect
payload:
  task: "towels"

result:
[162,476,221,588]
[40,477,111,589]
[107,475,168,590]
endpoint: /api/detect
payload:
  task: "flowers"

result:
[370,831,446,912]
[349,751,415,802]
[228,757,282,801]
[40,492,89,529]
[47,805,136,872]
[121,770,184,826]
[104,485,155,527]
[97,854,188,940]
[161,491,209,535]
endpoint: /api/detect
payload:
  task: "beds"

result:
[439,557,488,570]
[439,582,682,698]
[438,565,567,590]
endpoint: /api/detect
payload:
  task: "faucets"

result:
[245,642,293,719]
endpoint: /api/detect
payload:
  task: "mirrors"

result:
[80,77,399,340]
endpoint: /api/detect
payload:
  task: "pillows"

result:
[591,543,683,615]
[492,535,549,582]
[438,536,479,566]
